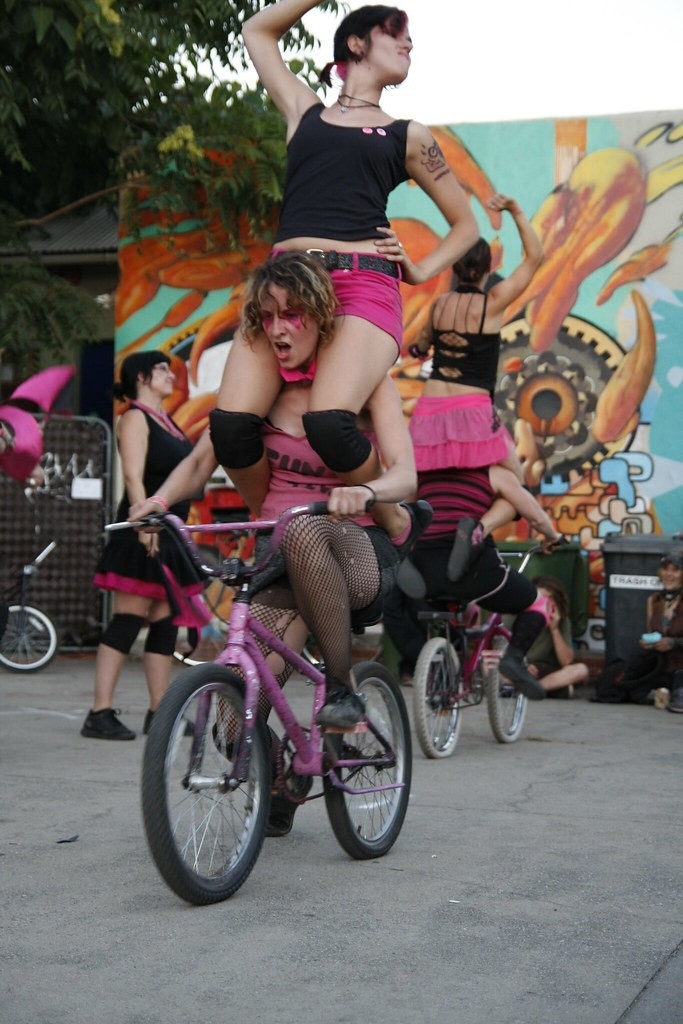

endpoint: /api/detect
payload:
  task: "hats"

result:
[659,546,683,569]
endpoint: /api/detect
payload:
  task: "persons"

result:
[79,351,210,741]
[209,0,480,563]
[380,193,571,700]
[0,397,46,489]
[127,250,418,837]
[499,577,587,699]
[628,550,683,713]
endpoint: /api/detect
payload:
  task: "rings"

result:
[399,242,402,248]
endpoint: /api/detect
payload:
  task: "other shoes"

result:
[667,684,683,712]
[497,652,546,701]
[588,685,630,704]
[384,500,433,563]
[400,671,413,687]
[396,559,425,599]
[448,515,482,582]
[547,685,574,698]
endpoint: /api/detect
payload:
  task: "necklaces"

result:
[338,95,380,113]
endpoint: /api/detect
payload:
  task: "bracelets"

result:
[145,496,169,512]
[351,484,376,507]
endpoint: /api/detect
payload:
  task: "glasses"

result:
[152,365,171,374]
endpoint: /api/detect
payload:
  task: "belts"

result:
[277,248,398,280]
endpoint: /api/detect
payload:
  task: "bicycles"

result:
[412,534,571,760]
[0,451,64,671]
[103,497,415,904]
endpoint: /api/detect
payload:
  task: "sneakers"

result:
[315,684,367,727]
[144,708,207,737]
[264,772,303,838]
[80,708,135,739]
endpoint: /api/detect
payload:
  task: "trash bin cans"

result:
[600,531,683,667]
[480,540,591,701]
